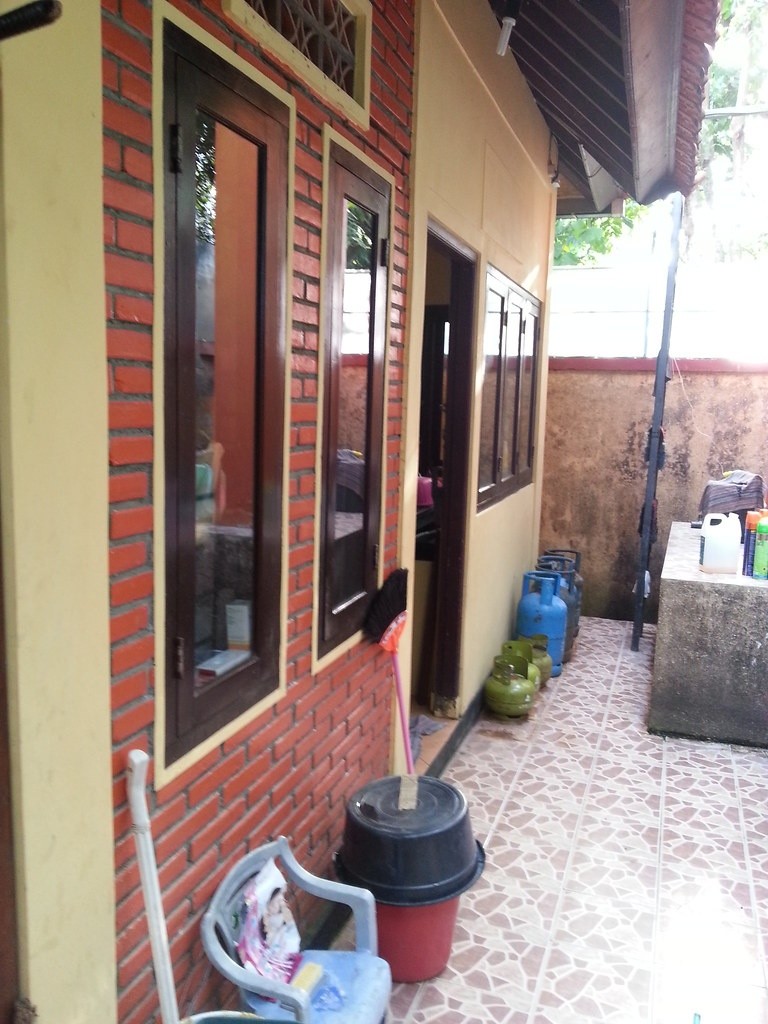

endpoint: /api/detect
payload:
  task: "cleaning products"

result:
[698,508,768,580]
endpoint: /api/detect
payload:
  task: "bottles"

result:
[758,509,768,521]
[752,517,768,581]
[698,512,742,574]
[742,510,762,576]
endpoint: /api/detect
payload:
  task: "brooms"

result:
[360,565,415,777]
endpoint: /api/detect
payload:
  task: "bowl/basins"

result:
[331,773,485,907]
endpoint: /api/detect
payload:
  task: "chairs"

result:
[199,836,393,1024]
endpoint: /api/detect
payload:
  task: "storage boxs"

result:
[226,599,253,650]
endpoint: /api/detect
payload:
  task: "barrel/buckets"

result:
[368,886,461,985]
